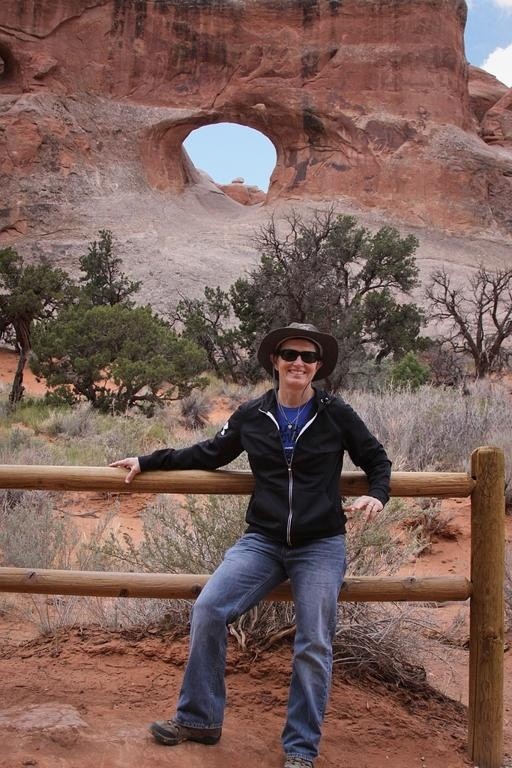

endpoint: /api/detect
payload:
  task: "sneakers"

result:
[283,755,314,768]
[149,718,222,746]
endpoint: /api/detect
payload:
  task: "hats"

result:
[257,322,338,382]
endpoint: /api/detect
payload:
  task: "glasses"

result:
[275,348,321,363]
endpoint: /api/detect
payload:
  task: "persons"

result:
[105,321,395,767]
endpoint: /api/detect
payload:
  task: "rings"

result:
[367,503,374,507]
